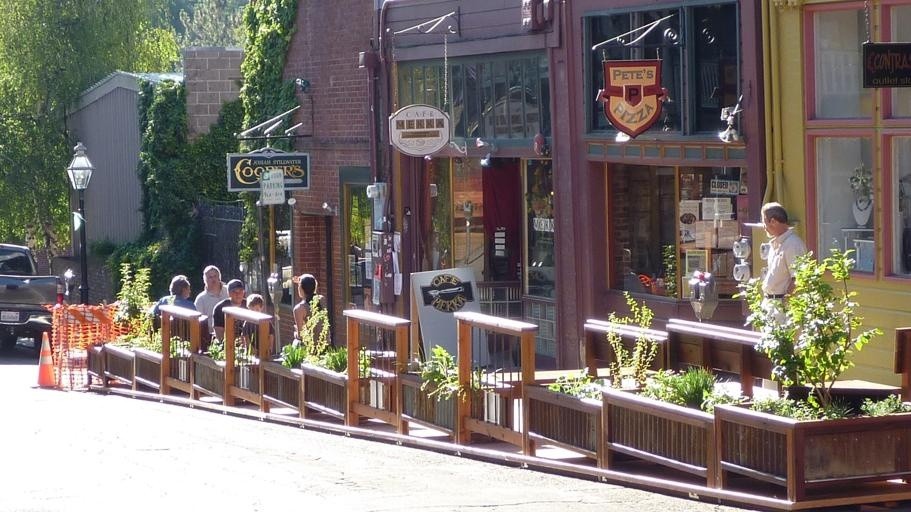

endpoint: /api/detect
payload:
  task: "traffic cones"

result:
[38,331,56,388]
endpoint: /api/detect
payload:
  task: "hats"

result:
[227,279,245,292]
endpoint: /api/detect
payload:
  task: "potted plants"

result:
[89,246,911,500]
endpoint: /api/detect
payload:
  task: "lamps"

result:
[321,202,339,217]
[477,138,497,165]
[717,95,744,144]
[295,78,309,93]
[287,198,297,211]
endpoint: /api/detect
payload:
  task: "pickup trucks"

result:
[1,242,64,360]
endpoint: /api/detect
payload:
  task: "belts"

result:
[765,294,784,299]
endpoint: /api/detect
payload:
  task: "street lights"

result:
[66,141,96,304]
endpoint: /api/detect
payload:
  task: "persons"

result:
[192,263,228,344]
[241,293,275,356]
[755,201,810,343]
[210,278,247,352]
[289,273,331,353]
[148,274,197,341]
